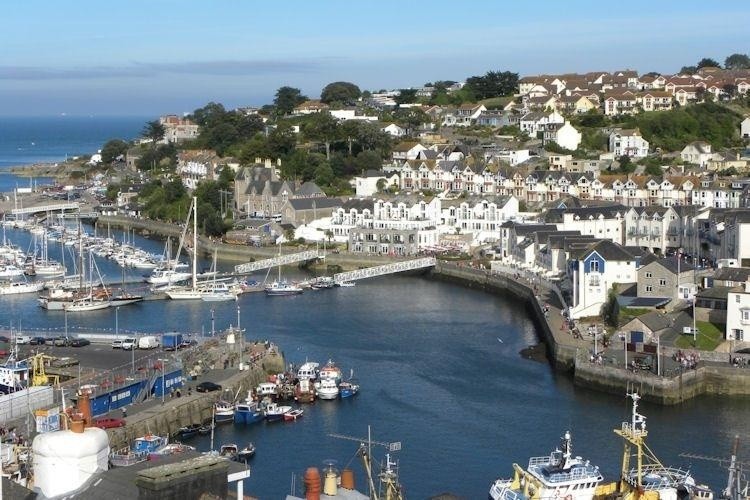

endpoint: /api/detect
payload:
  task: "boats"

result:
[182,422,216,437]
[109,421,195,466]
[214,359,360,424]
[487,394,750,500]
[220,442,255,463]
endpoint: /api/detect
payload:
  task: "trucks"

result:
[162,332,184,349]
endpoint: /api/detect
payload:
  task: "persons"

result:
[632,360,640,372]
[543,303,551,321]
[224,356,234,370]
[171,387,181,398]
[0,426,24,446]
[733,355,746,368]
[673,349,697,370]
[265,339,269,350]
[123,407,127,417]
[590,353,602,365]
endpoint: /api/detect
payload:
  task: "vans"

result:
[122,336,158,350]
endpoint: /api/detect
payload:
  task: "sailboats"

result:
[0,188,335,311]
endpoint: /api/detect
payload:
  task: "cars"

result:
[96,417,126,428]
[112,340,122,349]
[181,340,197,348]
[197,381,222,393]
[16,335,90,347]
